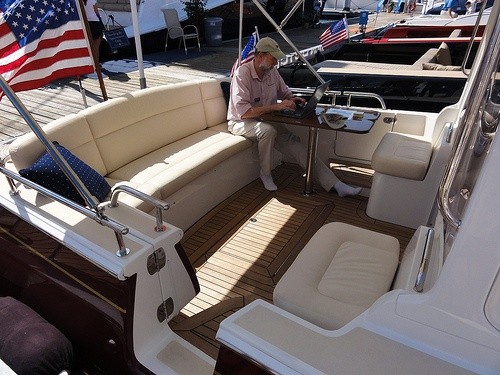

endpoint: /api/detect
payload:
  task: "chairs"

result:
[161,9,202,56]
[366,123,456,230]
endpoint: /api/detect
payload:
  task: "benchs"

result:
[10,77,281,232]
[311,60,413,71]
[273,222,435,331]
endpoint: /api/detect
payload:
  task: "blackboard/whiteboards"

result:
[104,27,130,49]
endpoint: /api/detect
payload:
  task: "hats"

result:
[254,37,287,61]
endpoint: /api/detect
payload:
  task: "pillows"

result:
[435,66,461,71]
[422,63,443,70]
[18,141,111,206]
[436,42,452,65]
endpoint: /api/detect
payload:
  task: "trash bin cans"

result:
[204,17,223,46]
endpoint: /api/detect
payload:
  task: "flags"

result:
[0,0,96,102]
[229,32,256,77]
[319,18,348,49]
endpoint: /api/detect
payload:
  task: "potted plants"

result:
[302,16,311,29]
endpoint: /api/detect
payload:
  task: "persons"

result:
[383,0,468,17]
[227,37,364,199]
[82,0,104,68]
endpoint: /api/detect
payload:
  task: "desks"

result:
[263,105,381,196]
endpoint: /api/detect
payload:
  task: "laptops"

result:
[273,80,330,119]
